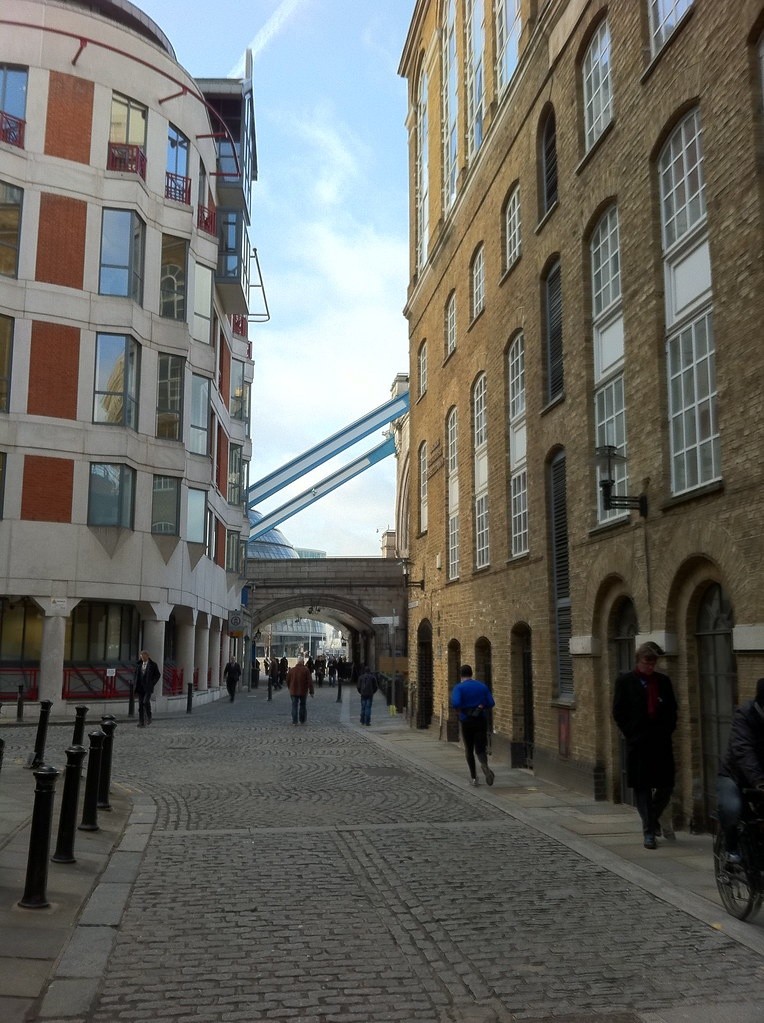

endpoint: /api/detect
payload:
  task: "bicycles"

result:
[710,788,763,921]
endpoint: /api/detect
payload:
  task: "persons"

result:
[252,659,260,668]
[402,670,408,709]
[287,660,314,725]
[714,678,764,873]
[357,667,378,726]
[223,656,241,703]
[133,650,161,728]
[305,654,347,688]
[451,665,495,786]
[264,655,288,690]
[612,641,678,850]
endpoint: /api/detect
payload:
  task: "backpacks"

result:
[361,677,372,697]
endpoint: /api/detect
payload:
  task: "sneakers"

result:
[644,821,662,849]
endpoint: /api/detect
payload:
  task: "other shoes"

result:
[471,777,479,787]
[725,852,745,873]
[361,721,371,726]
[483,765,496,787]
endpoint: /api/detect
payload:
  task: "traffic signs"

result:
[371,616,399,635]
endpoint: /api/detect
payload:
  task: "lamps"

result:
[395,557,425,592]
[253,627,261,641]
[586,445,649,521]
[436,551,442,571]
